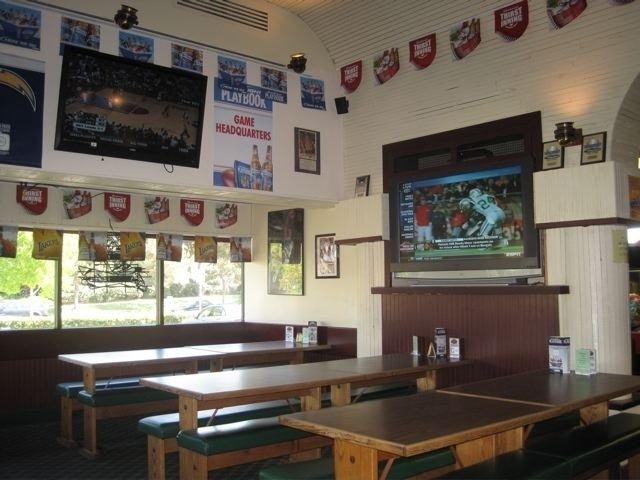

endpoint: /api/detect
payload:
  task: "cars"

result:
[0,298,51,316]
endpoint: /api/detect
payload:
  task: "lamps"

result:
[287,54,307,73]
[114,5,138,29]
[554,121,583,146]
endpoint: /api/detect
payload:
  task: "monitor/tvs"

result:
[53,43,209,169]
[383,153,543,285]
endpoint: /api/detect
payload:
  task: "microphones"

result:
[334,96,348,114]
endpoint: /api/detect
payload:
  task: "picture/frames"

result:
[314,233,339,279]
[294,127,320,174]
[354,175,370,197]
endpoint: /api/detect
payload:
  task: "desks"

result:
[57,340,640,480]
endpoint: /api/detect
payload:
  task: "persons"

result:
[403,174,524,253]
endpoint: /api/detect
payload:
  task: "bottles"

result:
[383,48,399,67]
[250,144,273,192]
[157,233,174,261]
[462,18,480,39]
[230,237,244,262]
[78,231,97,260]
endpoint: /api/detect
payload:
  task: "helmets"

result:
[458,198,471,210]
[469,188,482,202]
